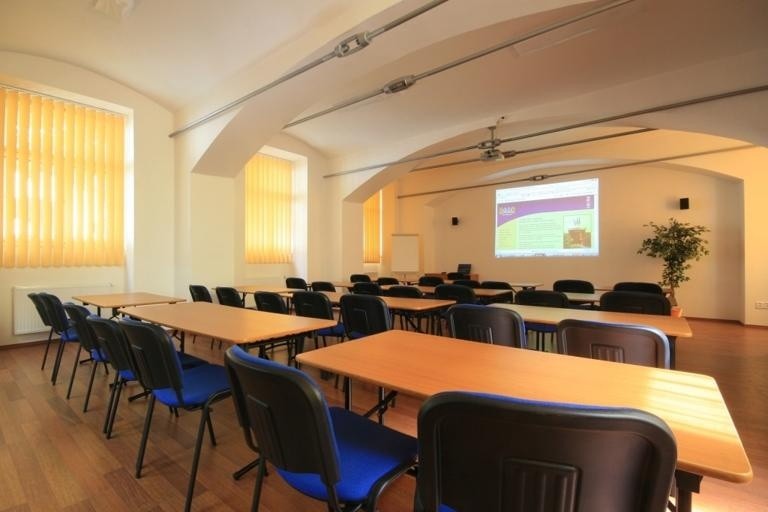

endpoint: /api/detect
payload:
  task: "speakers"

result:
[680,198,689,209]
[452,217,457,225]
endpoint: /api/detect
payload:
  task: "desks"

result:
[325,281,513,298]
[117,301,339,345]
[71,292,185,317]
[564,292,602,302]
[294,329,754,512]
[487,302,693,370]
[211,283,457,338]
[508,282,544,290]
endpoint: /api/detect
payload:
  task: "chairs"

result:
[552,279,594,295]
[189,284,215,351]
[286,277,308,315]
[387,285,423,299]
[63,300,128,414]
[340,294,392,391]
[354,282,384,296]
[87,315,175,437]
[514,289,570,352]
[558,318,671,369]
[447,305,527,356]
[411,392,679,512]
[312,281,336,293]
[41,291,111,398]
[447,272,465,281]
[377,277,399,286]
[292,291,345,378]
[216,286,244,352]
[435,283,476,304]
[481,281,512,290]
[122,318,233,512]
[28,291,93,382]
[222,343,421,512]
[254,291,287,359]
[599,290,672,316]
[612,282,664,295]
[350,274,372,283]
[452,280,480,289]
[418,276,444,287]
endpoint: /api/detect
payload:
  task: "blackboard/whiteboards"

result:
[390,236,420,272]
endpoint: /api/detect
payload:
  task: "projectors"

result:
[480,148,502,161]
